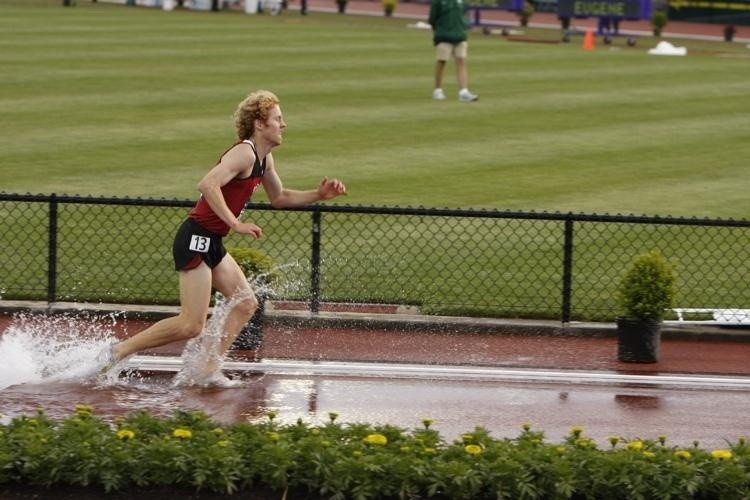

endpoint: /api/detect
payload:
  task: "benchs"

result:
[465,5,526,29]
[555,10,641,37]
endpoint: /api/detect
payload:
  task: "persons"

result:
[86,90,348,392]
[428,0,483,103]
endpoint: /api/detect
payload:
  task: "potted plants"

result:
[612,246,677,364]
[515,2,536,29]
[381,0,394,17]
[223,244,281,351]
[649,8,667,36]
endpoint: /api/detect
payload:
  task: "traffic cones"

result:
[580,25,595,50]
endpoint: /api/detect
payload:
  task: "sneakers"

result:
[432,87,446,101]
[457,88,478,102]
